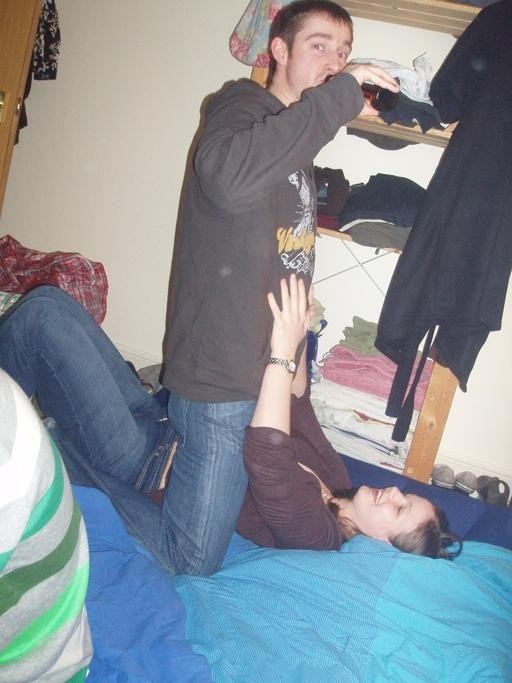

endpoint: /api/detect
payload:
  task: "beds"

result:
[73,452,512,683]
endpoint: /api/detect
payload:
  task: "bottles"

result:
[325,74,400,114]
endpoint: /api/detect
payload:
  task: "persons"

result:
[0,272,463,562]
[0,363,99,683]
[99,1,399,575]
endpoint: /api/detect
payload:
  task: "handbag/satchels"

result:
[0,235,108,325]
[313,166,349,217]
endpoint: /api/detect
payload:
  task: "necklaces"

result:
[321,486,350,512]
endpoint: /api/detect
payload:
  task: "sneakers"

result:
[431,465,512,511]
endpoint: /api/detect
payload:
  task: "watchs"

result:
[266,354,296,372]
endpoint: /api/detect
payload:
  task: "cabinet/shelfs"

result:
[1,0,45,210]
[251,0,486,484]
